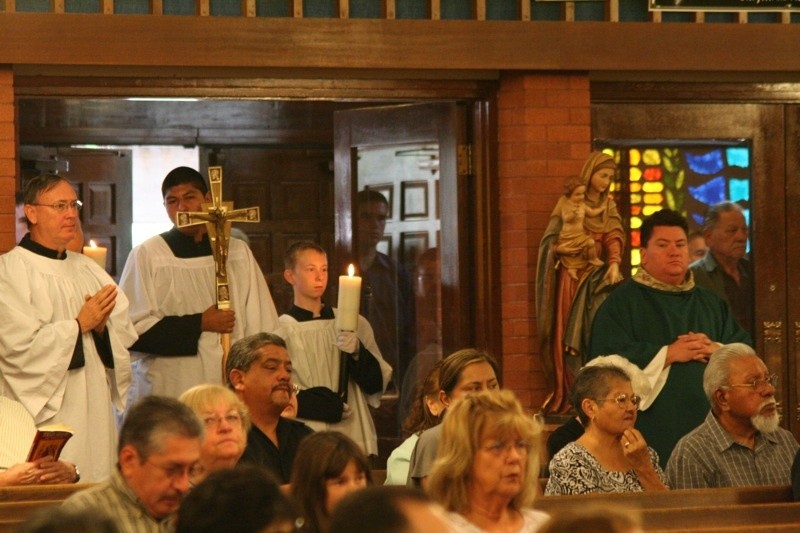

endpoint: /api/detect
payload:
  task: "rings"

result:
[625,441,630,448]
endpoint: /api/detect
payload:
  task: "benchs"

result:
[0,475,800,533]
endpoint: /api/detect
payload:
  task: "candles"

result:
[336,264,362,331]
[83,239,107,269]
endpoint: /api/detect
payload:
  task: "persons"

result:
[61,395,208,533]
[0,455,80,486]
[688,201,756,353]
[544,354,672,498]
[118,167,289,430]
[226,332,317,488]
[187,209,248,278]
[383,347,552,533]
[176,384,250,472]
[533,150,626,416]
[663,342,800,489]
[354,191,403,387]
[17,507,120,533]
[0,173,139,484]
[175,466,300,533]
[286,430,456,532]
[589,208,753,472]
[277,238,393,457]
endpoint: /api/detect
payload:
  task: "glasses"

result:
[144,459,205,478]
[595,394,641,409]
[726,376,778,392]
[204,413,242,427]
[33,200,83,211]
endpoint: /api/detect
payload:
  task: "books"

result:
[25,423,74,462]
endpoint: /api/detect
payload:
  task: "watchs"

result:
[71,464,80,483]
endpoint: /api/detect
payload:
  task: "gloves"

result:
[343,403,351,419]
[338,331,359,353]
